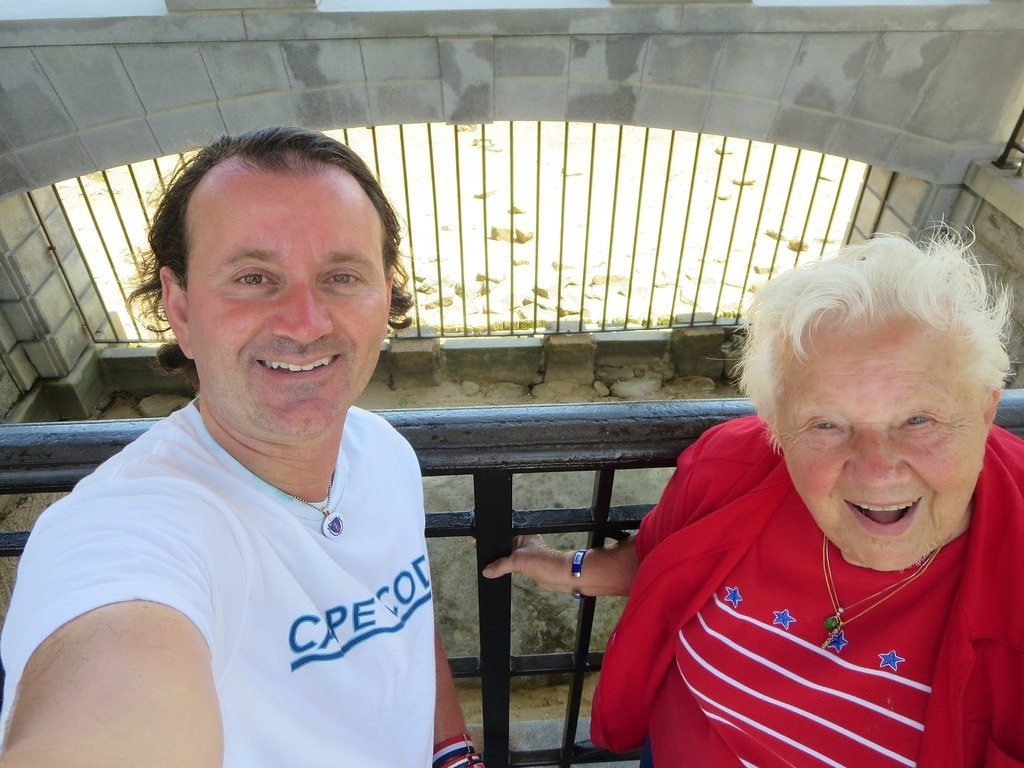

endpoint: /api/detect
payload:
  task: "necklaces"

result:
[224,449,345,540]
[820,533,946,654]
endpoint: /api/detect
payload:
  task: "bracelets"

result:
[433,733,476,768]
[438,753,485,768]
[571,549,588,599]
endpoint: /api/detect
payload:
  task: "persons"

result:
[0,125,486,768]
[483,210,1024,768]
